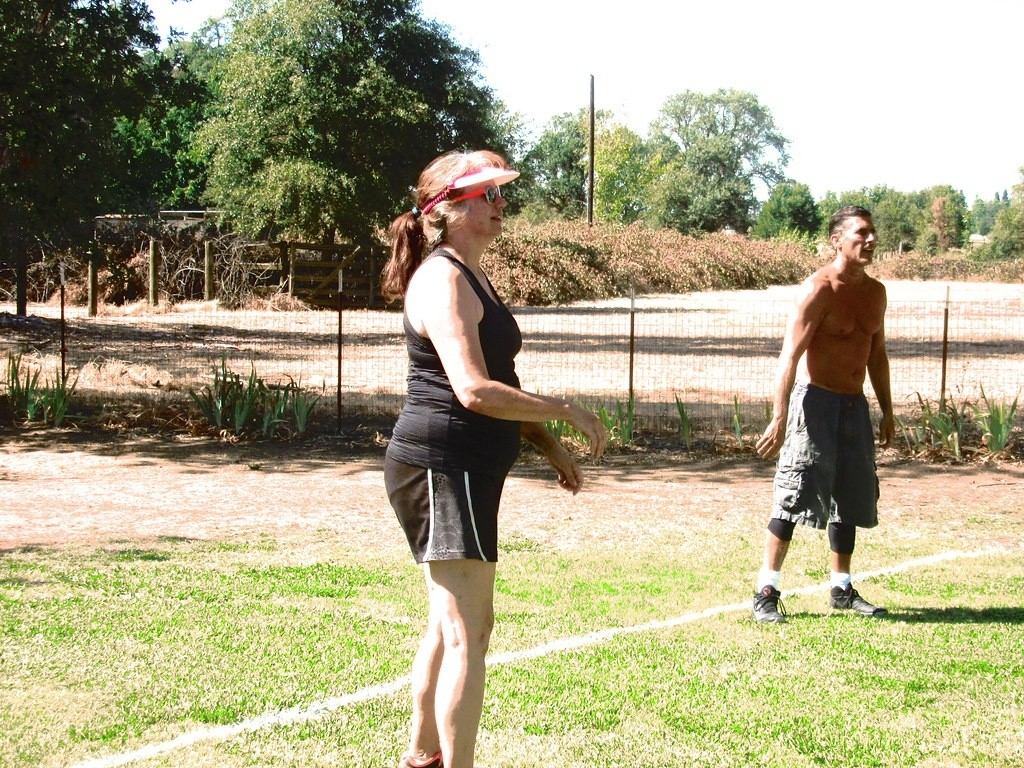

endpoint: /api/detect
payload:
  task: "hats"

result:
[420,165,520,216]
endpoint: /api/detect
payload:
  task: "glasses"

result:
[446,185,501,205]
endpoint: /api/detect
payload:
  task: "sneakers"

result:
[754,585,786,624]
[399,749,444,768]
[830,582,889,616]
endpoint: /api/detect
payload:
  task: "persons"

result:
[382,150,608,768]
[753,206,896,622]
